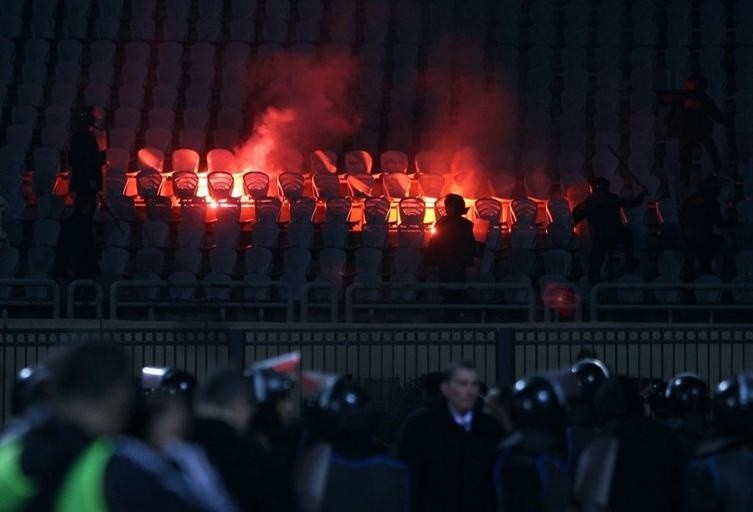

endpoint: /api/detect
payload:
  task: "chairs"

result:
[0,1,753,320]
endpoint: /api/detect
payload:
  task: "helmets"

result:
[716,374,752,425]
[255,370,290,404]
[329,380,364,413]
[666,375,712,416]
[698,176,722,196]
[570,361,608,400]
[138,364,194,399]
[16,362,56,406]
[591,178,610,195]
[511,375,558,428]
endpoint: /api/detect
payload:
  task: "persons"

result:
[429,194,480,314]
[679,175,736,271]
[68,110,103,241]
[658,74,730,171]
[572,177,646,274]
[0,339,753,512]
